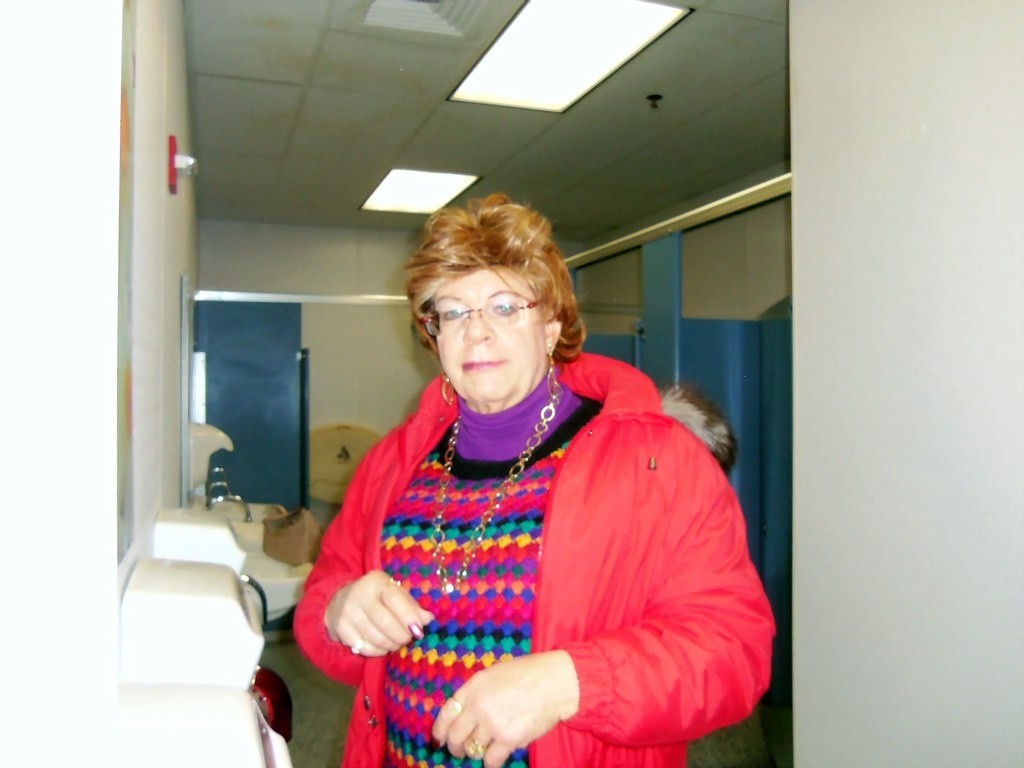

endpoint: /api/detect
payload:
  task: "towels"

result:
[264,508,325,566]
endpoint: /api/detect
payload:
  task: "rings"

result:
[467,739,486,756]
[351,637,364,654]
[390,577,400,587]
[447,698,463,713]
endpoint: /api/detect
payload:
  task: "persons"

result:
[294,194,776,768]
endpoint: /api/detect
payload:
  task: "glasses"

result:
[418,300,539,336]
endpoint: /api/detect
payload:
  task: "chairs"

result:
[308,425,385,503]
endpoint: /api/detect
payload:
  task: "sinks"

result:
[152,508,315,621]
[190,501,286,520]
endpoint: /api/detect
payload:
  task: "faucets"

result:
[205,465,252,522]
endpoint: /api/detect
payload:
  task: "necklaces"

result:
[431,385,562,594]
